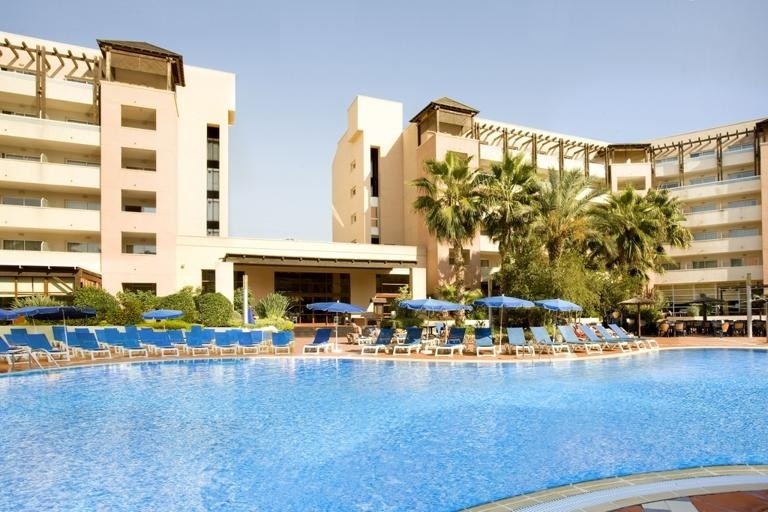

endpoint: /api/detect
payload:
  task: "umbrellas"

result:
[16,307,39,325]
[534,298,583,343]
[687,297,724,321]
[306,300,367,352]
[399,296,473,354]
[27,305,96,355]
[475,294,535,353]
[0,309,18,326]
[618,297,655,339]
[143,310,183,330]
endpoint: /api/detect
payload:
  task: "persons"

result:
[369,328,381,337]
[436,315,448,337]
[347,323,362,345]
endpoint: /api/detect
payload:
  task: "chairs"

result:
[351,323,656,357]
[303,329,332,354]
[1,325,295,373]
[625,317,768,338]
